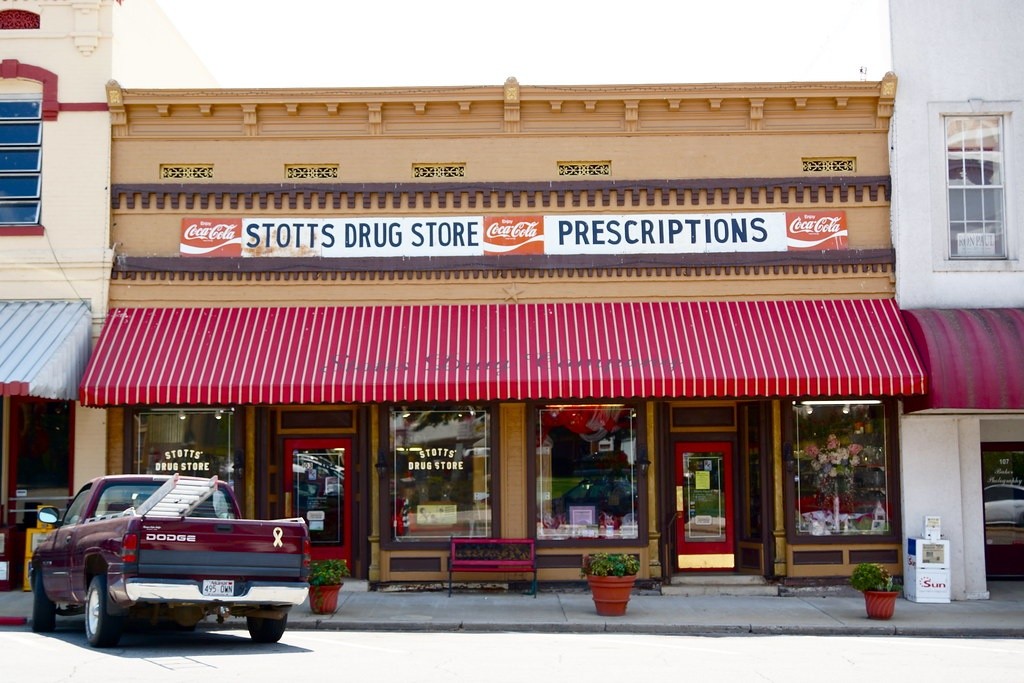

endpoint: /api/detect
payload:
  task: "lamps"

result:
[842,405,851,414]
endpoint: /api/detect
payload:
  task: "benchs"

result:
[447,534,537,598]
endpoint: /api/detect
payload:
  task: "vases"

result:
[586,575,637,617]
[832,478,844,533]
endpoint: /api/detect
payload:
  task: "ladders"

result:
[137,473,217,518]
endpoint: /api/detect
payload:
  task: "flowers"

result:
[801,433,863,514]
[580,553,640,577]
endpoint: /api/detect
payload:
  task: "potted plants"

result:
[849,564,903,621]
[308,559,352,614]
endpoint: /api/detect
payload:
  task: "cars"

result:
[983,485,1024,527]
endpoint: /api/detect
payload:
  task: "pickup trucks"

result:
[28,473,311,647]
